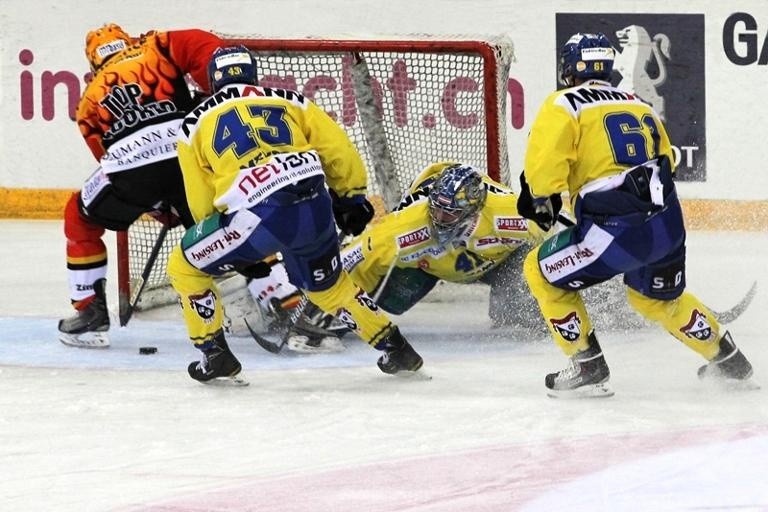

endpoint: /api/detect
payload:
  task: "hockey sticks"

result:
[244,296,309,354]
[119,224,170,327]
[555,214,756,323]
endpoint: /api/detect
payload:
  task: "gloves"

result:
[332,189,375,236]
[239,260,274,280]
[516,171,563,231]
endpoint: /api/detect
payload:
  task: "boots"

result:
[546,328,610,392]
[223,287,353,349]
[697,329,754,380]
[377,326,423,373]
[58,295,110,332]
[188,329,242,381]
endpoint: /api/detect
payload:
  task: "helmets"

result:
[429,163,487,233]
[207,43,258,96]
[560,31,615,78]
[84,23,133,72]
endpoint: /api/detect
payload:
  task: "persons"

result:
[218,159,558,353]
[166,46,433,388]
[57,20,228,351]
[517,29,761,401]
